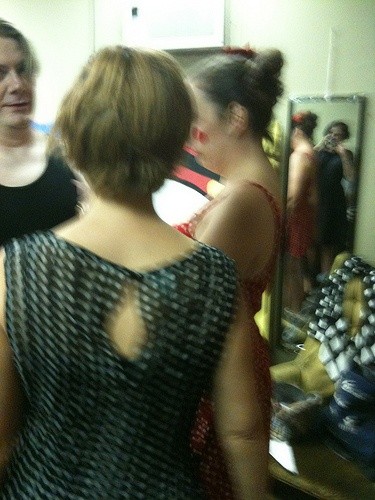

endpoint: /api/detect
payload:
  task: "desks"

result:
[268,344,375,500]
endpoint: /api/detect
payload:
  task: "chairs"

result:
[269,252,375,396]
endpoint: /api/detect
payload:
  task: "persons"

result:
[0,48,275,500]
[283,106,359,329]
[152,48,285,500]
[0,16,85,250]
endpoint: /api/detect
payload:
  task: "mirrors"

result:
[268,92,366,362]
